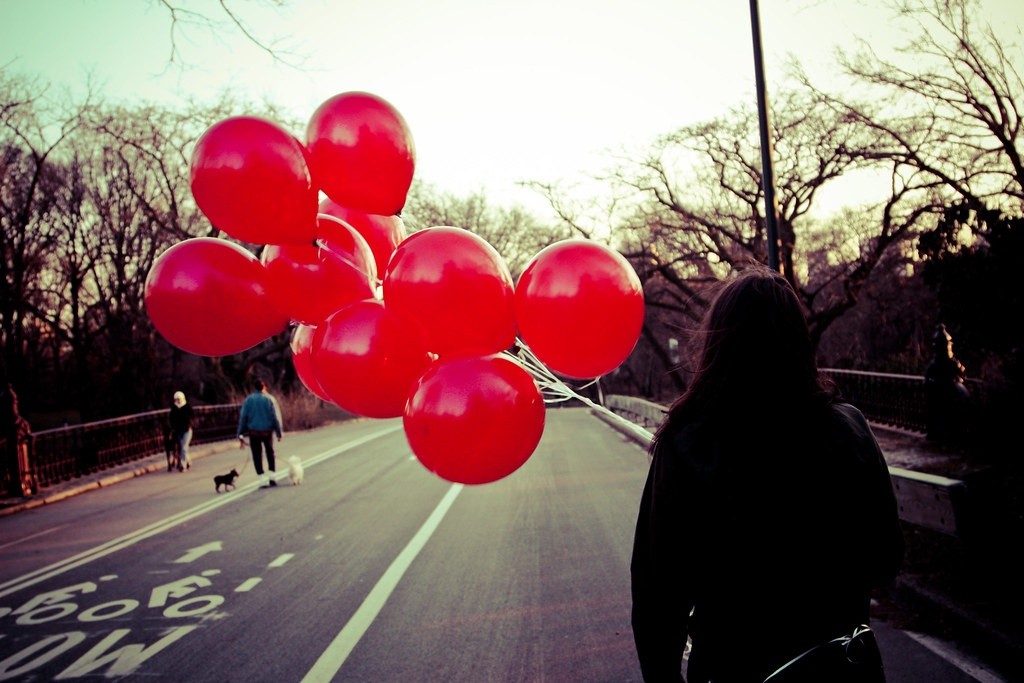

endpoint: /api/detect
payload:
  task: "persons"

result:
[629,267,906,683]
[169,392,193,473]
[238,378,283,490]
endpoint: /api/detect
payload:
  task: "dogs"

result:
[160,425,191,472]
[214,468,239,494]
[287,456,304,486]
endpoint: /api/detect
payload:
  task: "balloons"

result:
[145,92,645,485]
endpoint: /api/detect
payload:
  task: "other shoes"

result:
[260,484,268,488]
[269,480,277,486]
